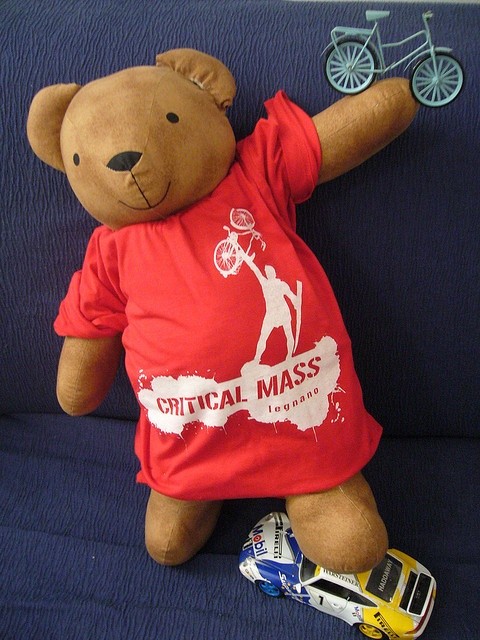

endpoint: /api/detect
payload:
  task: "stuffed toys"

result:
[24,47,420,577]
